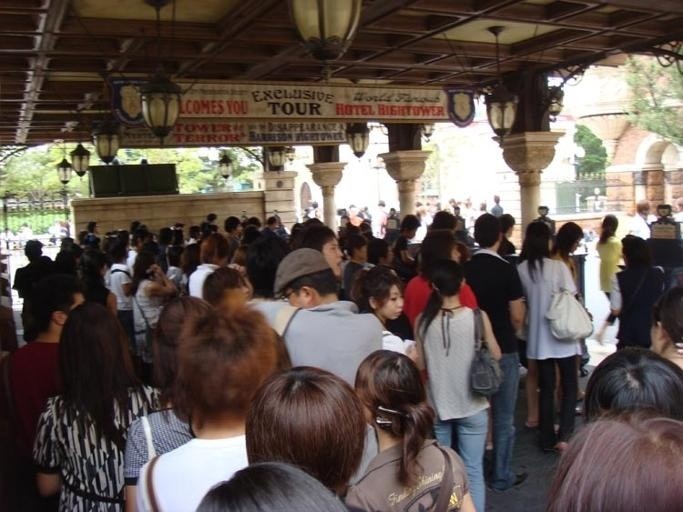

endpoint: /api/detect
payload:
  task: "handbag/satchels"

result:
[469,305,508,397]
[543,260,594,341]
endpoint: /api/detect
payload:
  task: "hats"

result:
[272,246,330,300]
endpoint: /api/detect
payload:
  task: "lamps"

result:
[269,145,287,175]
[92,59,119,165]
[548,70,563,125]
[292,1,363,84]
[56,102,91,185]
[350,122,370,159]
[138,2,183,141]
[221,147,235,182]
[486,24,520,145]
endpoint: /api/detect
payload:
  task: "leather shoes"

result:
[484,472,528,493]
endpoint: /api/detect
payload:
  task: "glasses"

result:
[279,284,306,302]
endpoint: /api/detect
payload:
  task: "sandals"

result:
[485,390,598,458]
